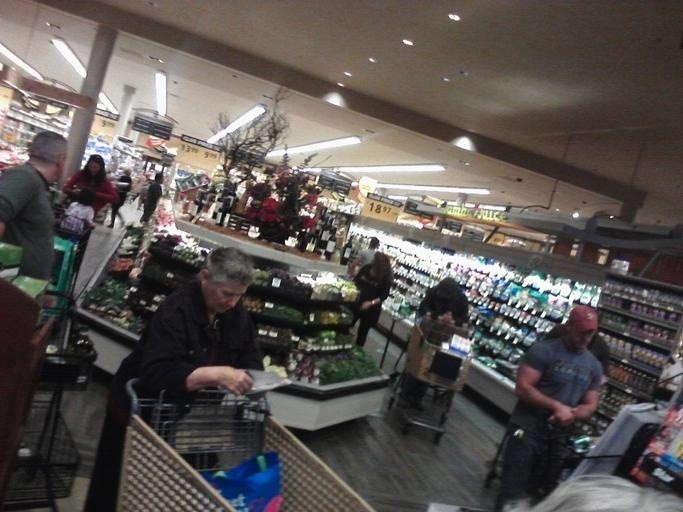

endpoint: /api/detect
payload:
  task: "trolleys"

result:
[388,315,474,445]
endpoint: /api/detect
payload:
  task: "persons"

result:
[82,246,266,512]
[419,277,468,326]
[61,155,120,221]
[107,168,132,228]
[0,130,68,280]
[352,237,394,347]
[492,304,611,511]
[137,171,164,223]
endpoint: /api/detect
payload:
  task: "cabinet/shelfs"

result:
[573,274,683,443]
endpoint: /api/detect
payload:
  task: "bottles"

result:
[549,279,682,435]
[188,191,205,224]
[216,198,233,228]
[316,206,337,258]
[340,234,355,265]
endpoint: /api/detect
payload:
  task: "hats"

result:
[570,305,598,332]
